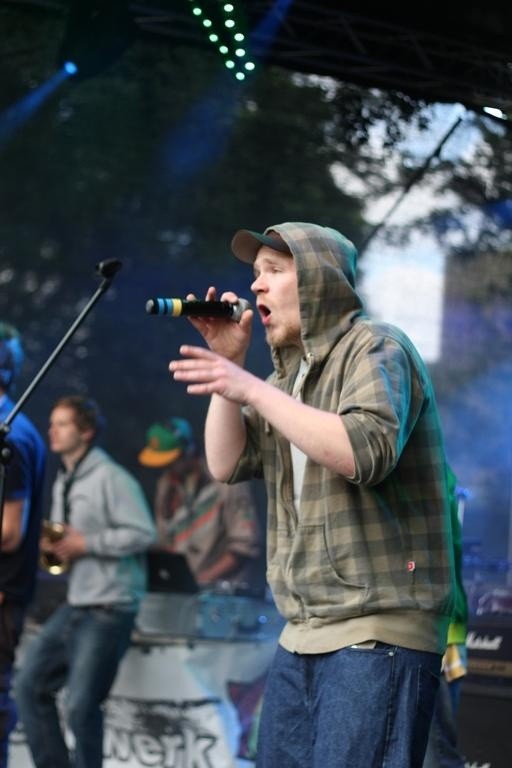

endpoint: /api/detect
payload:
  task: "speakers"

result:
[457,682,512,767]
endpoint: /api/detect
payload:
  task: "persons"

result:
[415,457,471,766]
[166,220,455,766]
[15,396,159,767]
[1,325,50,768]
[137,416,259,597]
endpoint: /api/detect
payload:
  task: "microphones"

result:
[146,296,251,322]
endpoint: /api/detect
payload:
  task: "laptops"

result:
[147,550,199,595]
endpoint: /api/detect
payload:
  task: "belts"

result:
[80,604,136,618]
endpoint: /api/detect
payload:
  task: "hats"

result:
[229,228,293,266]
[137,416,193,469]
